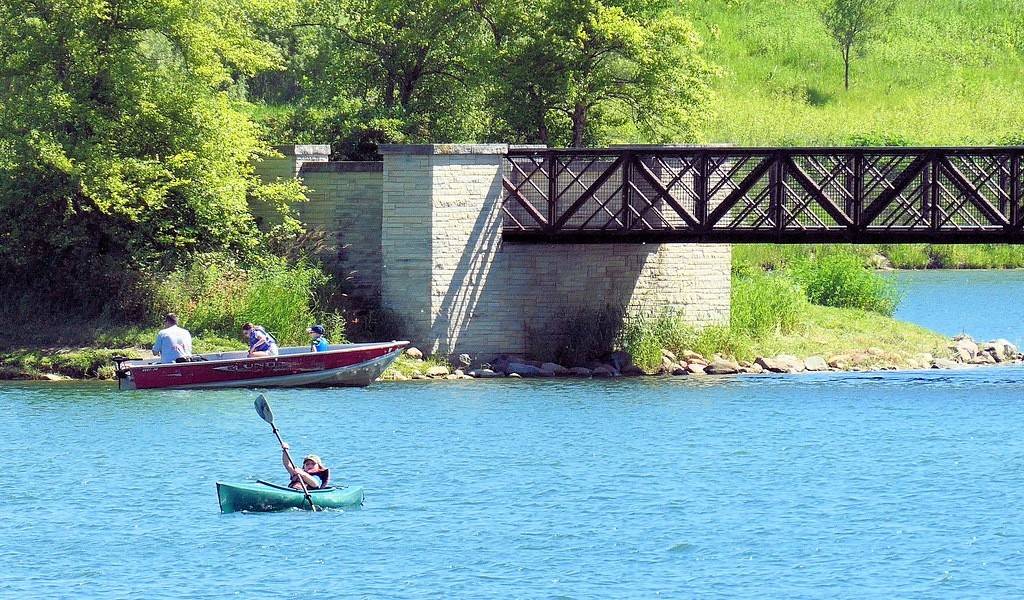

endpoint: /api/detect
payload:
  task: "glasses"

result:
[245,330,250,335]
[310,331,316,334]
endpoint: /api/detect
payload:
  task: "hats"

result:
[307,325,324,334]
[303,455,321,465]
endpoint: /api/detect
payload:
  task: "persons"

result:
[242,323,278,358]
[309,325,329,353]
[152,312,191,364]
[281,442,329,491]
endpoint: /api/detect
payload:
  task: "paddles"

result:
[255,394,323,516]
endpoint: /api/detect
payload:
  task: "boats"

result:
[111,340,411,390]
[216,479,366,515]
